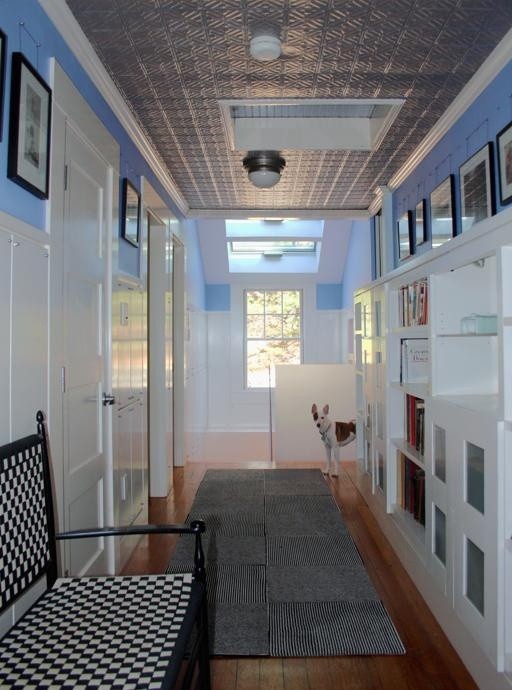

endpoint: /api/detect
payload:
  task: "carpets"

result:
[165,468,407,659]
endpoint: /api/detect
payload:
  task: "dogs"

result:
[311,402,356,478]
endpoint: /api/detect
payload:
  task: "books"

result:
[396,276,428,530]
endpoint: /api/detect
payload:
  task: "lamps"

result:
[243,150,285,188]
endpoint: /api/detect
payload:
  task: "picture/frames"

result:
[6,51,52,200]
[122,177,140,247]
[396,120,512,261]
[0,27,8,141]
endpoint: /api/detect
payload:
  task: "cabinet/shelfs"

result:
[353,204,512,678]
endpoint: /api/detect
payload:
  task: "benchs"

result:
[0,410,210,690]
